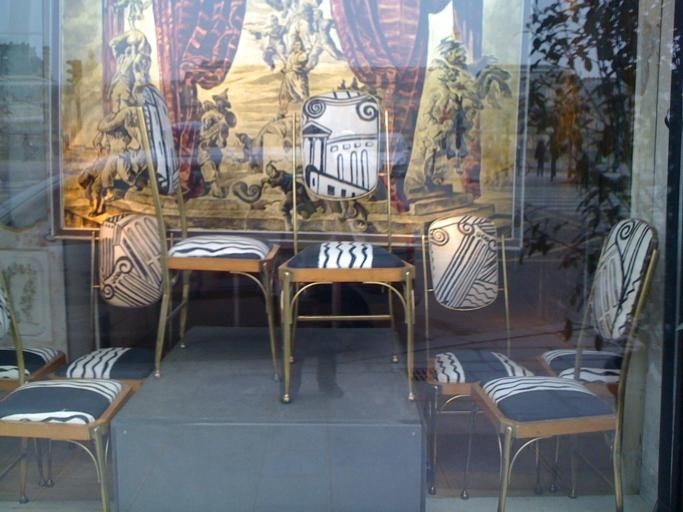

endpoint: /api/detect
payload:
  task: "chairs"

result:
[461,219,659,509]
[45,213,177,390]
[277,88,417,403]
[540,348,624,492]
[135,84,280,381]
[1,271,134,511]
[1,344,66,485]
[419,215,539,494]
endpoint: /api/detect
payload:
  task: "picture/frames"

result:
[49,1,532,254]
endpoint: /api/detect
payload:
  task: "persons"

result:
[95,72,182,198]
[186,88,235,200]
[275,35,324,106]
[261,162,326,234]
[259,17,292,72]
[235,131,263,168]
